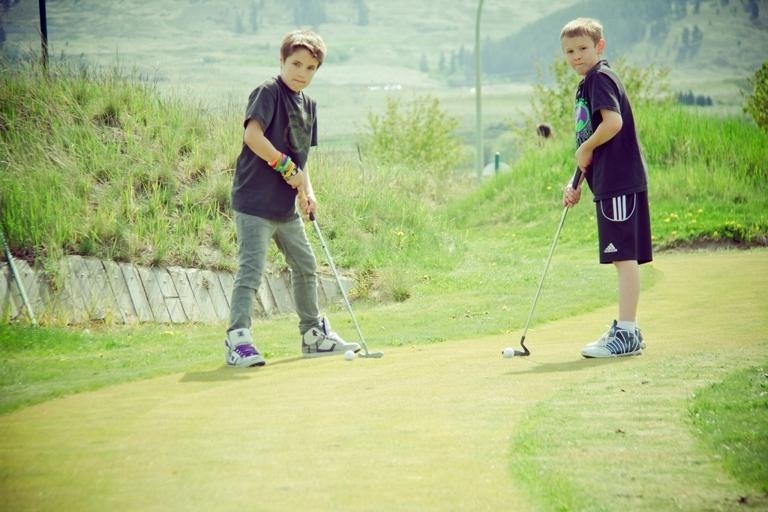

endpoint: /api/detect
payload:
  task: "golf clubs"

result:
[503,167,582,357]
[297,184,383,359]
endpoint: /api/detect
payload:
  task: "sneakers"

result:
[582,319,647,359]
[301,316,361,359]
[224,327,265,368]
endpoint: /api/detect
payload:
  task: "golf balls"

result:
[504,347,515,358]
[344,350,354,360]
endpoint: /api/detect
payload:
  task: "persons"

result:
[537,124,554,139]
[225,29,361,367]
[560,17,652,358]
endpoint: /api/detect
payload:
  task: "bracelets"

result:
[268,150,299,178]
